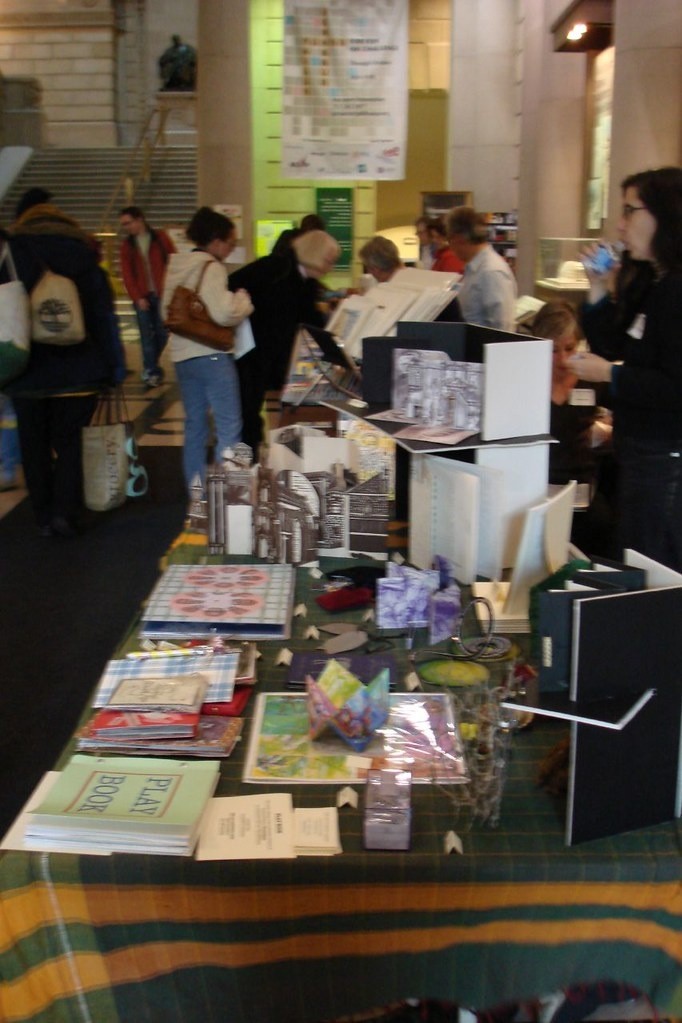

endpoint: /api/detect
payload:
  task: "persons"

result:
[426,218,467,275]
[416,215,435,271]
[557,167,682,573]
[160,206,254,503]
[446,206,518,333]
[527,299,619,557]
[228,213,352,466]
[160,34,197,90]
[0,187,126,536]
[117,207,178,385]
[359,236,465,323]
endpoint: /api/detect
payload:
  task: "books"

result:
[137,564,299,641]
[24,754,221,856]
[73,649,255,758]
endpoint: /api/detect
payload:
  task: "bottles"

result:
[580,239,626,277]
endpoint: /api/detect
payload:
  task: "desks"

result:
[0,513,682,1023]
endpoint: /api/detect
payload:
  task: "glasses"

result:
[623,204,651,219]
[364,263,377,273]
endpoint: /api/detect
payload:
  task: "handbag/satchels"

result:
[165,257,236,351]
[0,242,31,386]
[22,235,87,344]
[81,383,128,512]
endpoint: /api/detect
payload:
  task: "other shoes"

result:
[38,524,57,538]
[140,370,164,387]
[52,509,84,537]
